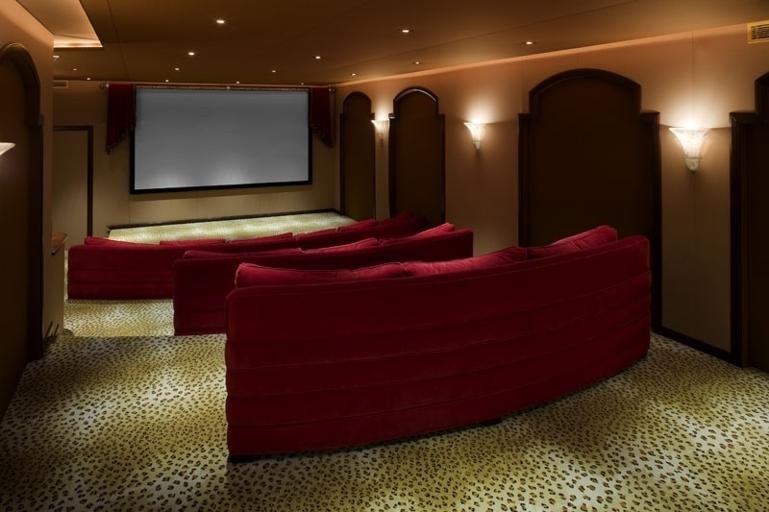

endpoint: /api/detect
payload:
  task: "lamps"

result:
[464,122,484,150]
[668,126,706,174]
[1,143,15,156]
[371,119,388,146]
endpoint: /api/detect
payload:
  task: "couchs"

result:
[171,222,477,337]
[222,225,655,465]
[66,211,416,305]
